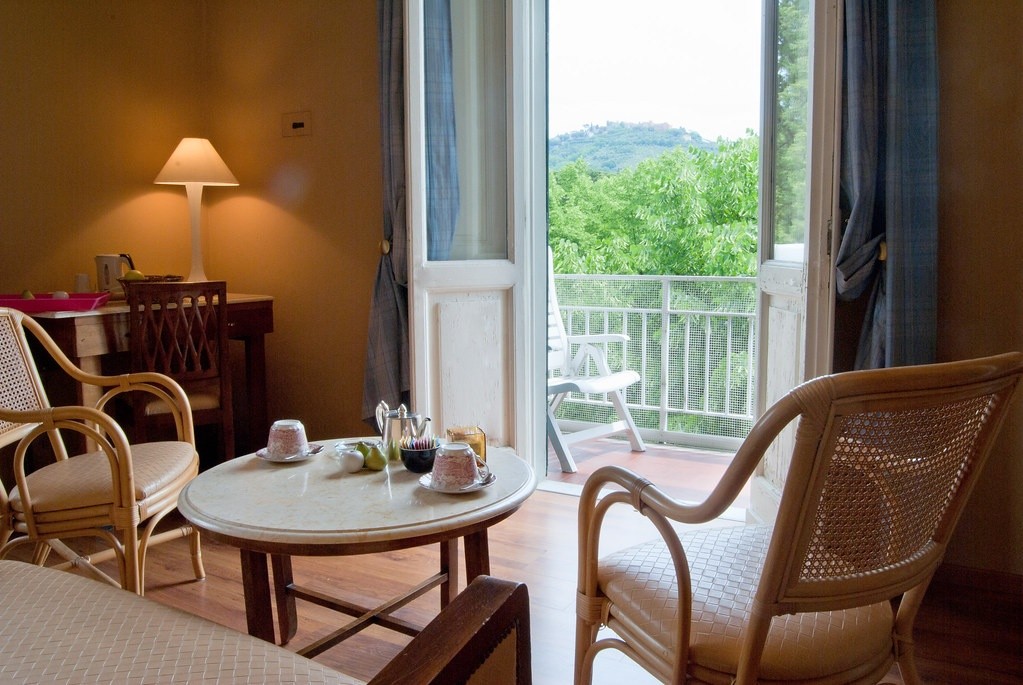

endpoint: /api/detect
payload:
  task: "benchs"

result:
[0,557,535,685]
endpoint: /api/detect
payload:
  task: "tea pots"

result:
[375,400,432,459]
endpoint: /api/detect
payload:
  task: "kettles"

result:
[95,253,136,292]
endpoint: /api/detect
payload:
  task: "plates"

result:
[256,444,324,462]
[418,471,496,493]
[334,440,380,454]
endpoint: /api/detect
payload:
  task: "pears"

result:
[124,270,145,281]
[21,289,35,299]
[354,441,388,471]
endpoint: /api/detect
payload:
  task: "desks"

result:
[24,292,274,458]
[175,434,539,658]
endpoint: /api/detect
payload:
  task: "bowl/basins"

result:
[399,446,438,473]
[117,275,183,304]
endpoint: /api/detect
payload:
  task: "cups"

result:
[430,442,489,488]
[265,419,309,459]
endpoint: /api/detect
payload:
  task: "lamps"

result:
[153,138,241,283]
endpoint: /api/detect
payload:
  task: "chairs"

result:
[574,351,1023,685]
[546,244,647,474]
[0,306,206,596]
[105,281,235,460]
